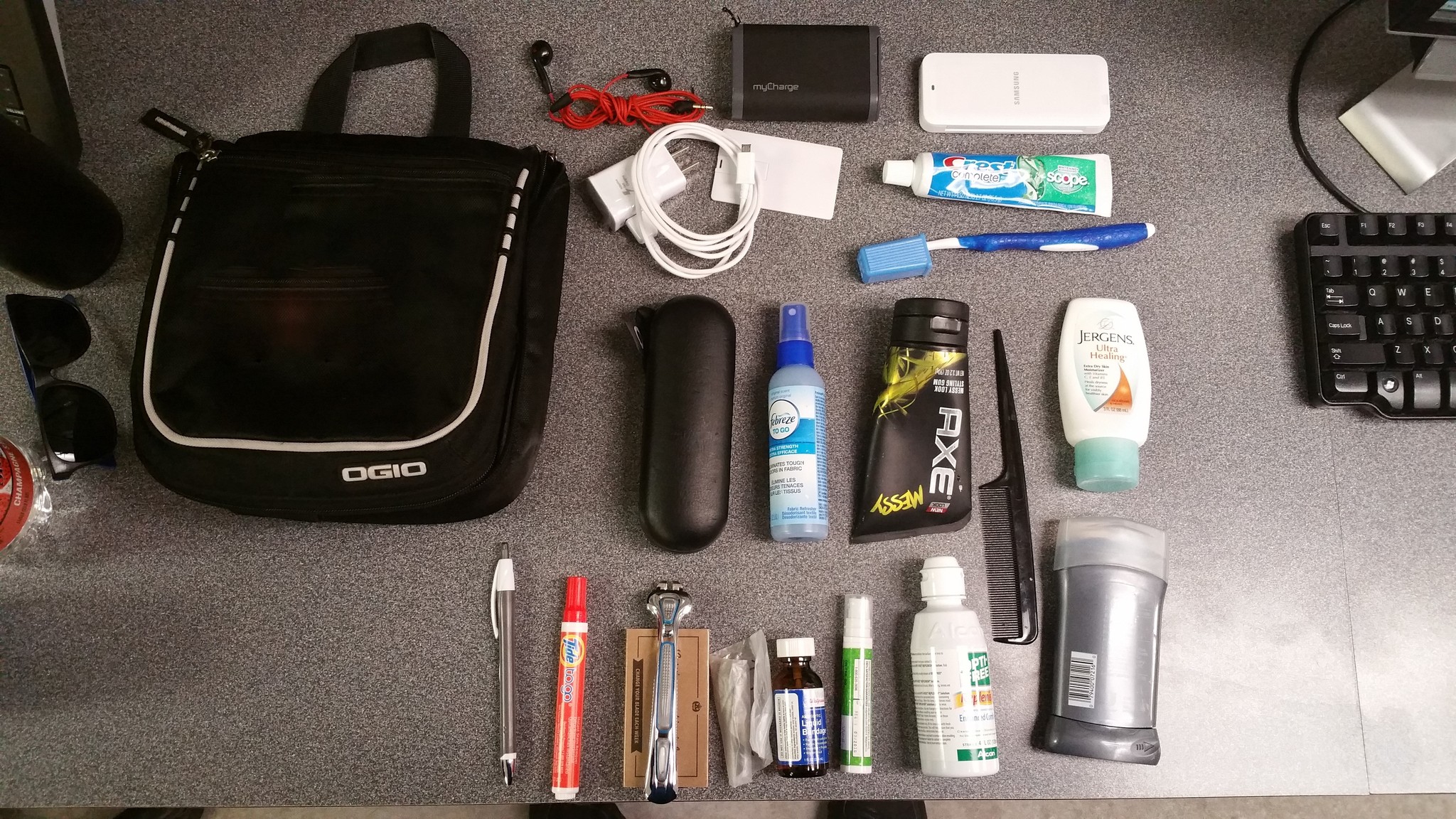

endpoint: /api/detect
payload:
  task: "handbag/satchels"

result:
[131,20,567,516]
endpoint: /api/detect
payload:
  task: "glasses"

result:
[5,289,119,480]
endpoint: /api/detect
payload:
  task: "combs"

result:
[976,328,1040,646]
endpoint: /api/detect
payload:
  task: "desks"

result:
[3,0,1456,804]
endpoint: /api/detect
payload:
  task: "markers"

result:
[550,575,590,803]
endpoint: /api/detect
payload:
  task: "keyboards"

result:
[1295,212,1456,419]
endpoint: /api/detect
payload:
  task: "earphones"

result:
[627,68,672,91]
[528,40,554,94]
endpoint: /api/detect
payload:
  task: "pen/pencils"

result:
[490,542,516,790]
